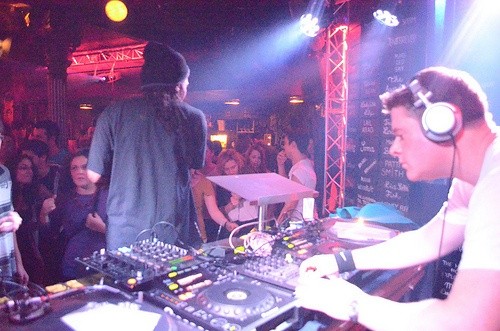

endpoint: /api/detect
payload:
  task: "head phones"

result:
[409,79,463,143]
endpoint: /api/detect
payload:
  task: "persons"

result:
[0,120,325,299]
[88,41,207,248]
[295,68,500,331]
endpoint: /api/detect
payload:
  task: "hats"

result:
[140,40,190,90]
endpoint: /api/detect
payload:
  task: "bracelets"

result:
[349,294,367,322]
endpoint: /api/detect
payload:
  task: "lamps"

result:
[372,0,404,27]
[300,6,335,36]
